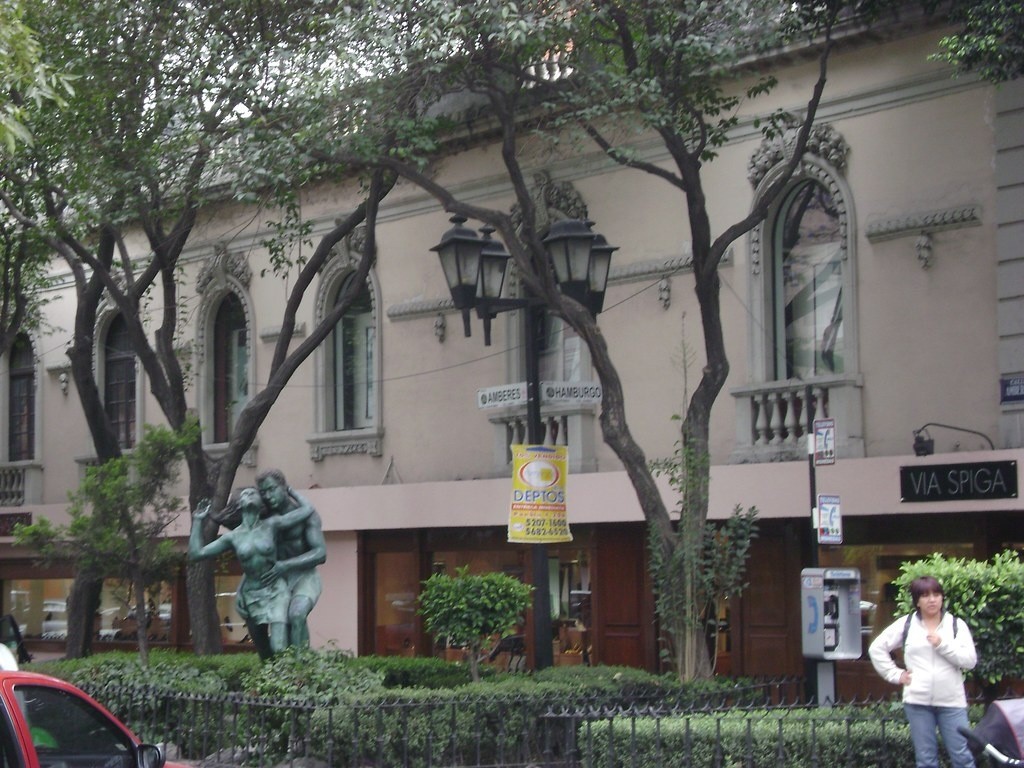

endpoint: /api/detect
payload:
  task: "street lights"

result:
[430,210,623,676]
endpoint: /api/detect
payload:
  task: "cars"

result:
[0,667,197,768]
[9,599,174,643]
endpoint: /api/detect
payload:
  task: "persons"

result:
[867,576,978,768]
[184,470,327,666]
